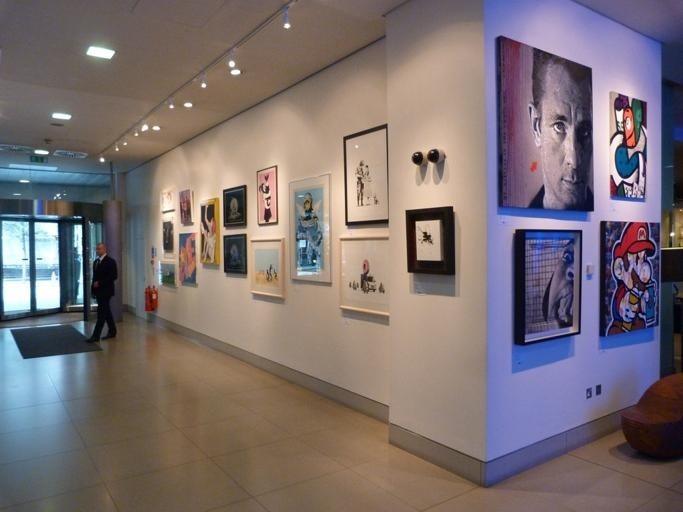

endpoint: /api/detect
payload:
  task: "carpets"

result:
[9,324,103,359]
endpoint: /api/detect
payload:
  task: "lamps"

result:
[93,1,294,164]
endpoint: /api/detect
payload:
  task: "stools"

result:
[620,370,682,464]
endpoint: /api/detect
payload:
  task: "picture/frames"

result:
[405,206,456,276]
[342,123,390,226]
[287,172,332,283]
[255,164,278,226]
[249,235,288,301]
[157,182,248,289]
[338,236,390,317]
[515,229,581,346]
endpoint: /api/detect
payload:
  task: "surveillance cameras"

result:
[412,152,427,167]
[427,149,445,164]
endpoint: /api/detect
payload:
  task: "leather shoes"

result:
[86,336,100,342]
[102,333,116,339]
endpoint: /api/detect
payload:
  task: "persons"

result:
[204,204,217,263]
[295,191,323,271]
[258,175,272,222]
[360,162,380,205]
[526,47,593,212]
[353,160,364,206]
[84,243,117,342]
[545,238,574,328]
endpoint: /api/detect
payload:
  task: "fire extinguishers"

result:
[145,286,151,311]
[151,285,156,311]
